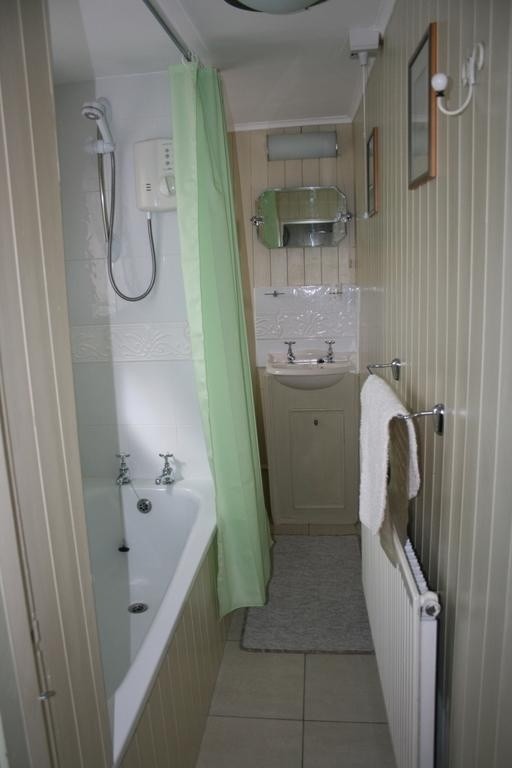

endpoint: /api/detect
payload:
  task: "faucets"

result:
[325,339,335,362]
[284,340,296,363]
[155,452,173,487]
[114,452,131,486]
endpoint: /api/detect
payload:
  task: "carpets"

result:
[240,535,375,653]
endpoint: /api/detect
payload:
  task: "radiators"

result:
[360,513,442,768]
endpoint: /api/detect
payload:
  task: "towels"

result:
[356,374,422,568]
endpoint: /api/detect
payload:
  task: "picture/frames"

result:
[367,127,379,217]
[409,21,437,189]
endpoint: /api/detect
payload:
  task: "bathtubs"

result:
[84,480,220,767]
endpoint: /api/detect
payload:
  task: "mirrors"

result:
[255,185,348,248]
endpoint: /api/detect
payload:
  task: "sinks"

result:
[265,362,351,389]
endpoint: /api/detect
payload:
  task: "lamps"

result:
[266,130,338,161]
[431,42,484,116]
